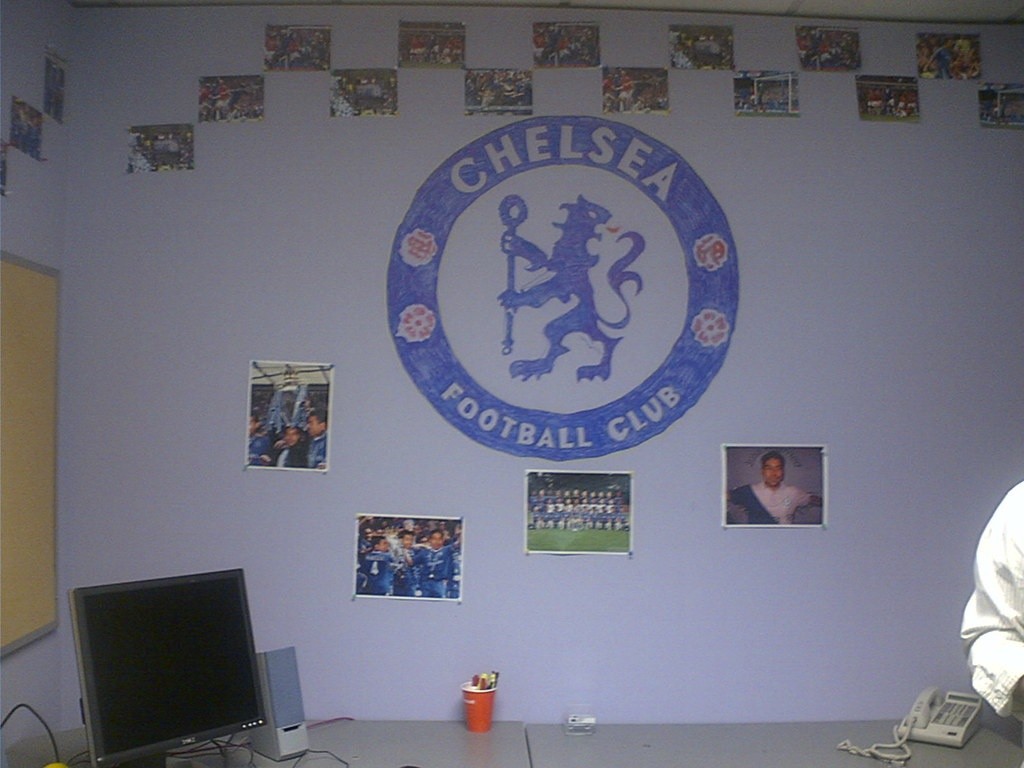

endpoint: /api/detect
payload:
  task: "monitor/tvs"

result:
[67,568,265,768]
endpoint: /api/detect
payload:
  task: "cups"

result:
[460,682,497,733]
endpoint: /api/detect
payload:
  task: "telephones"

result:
[897,687,984,747]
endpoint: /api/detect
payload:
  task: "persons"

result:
[959,481,1024,722]
[357,516,462,599]
[0,20,1024,196]
[727,452,821,524]
[250,389,328,469]
[529,487,629,532]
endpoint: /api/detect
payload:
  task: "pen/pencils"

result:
[472,671,499,691]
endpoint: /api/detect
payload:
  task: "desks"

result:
[3,719,1023,768]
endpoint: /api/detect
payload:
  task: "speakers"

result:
[252,646,308,759]
